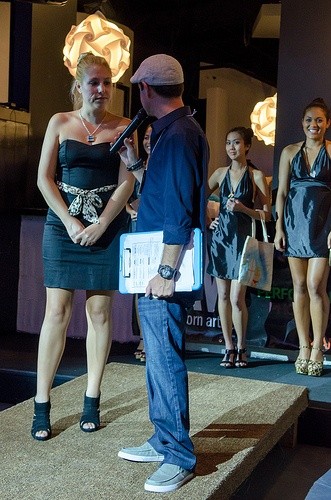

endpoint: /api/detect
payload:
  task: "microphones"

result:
[110,108,149,154]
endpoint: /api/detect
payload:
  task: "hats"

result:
[130,54,184,85]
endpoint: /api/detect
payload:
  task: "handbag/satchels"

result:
[237,209,275,291]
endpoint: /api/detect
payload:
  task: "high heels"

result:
[307,346,324,376]
[294,346,311,374]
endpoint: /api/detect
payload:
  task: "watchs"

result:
[156,264,176,280]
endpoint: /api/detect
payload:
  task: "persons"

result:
[273,96,331,376]
[124,114,153,362]
[110,53,211,493]
[207,124,272,369]
[30,52,140,441]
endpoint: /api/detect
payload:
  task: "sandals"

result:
[235,348,247,368]
[79,390,100,432]
[31,396,53,440]
[136,338,143,359]
[220,349,236,368]
[140,352,146,363]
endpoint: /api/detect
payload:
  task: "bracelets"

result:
[126,159,145,172]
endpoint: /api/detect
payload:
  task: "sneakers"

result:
[118,442,164,462]
[144,463,193,492]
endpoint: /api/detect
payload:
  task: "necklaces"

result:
[78,109,108,141]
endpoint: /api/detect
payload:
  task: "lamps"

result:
[250,92,278,146]
[63,10,131,83]
